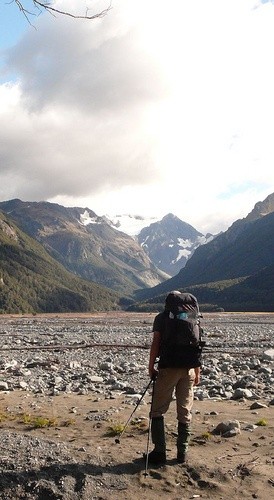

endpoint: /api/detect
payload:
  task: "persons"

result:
[144,291,200,463]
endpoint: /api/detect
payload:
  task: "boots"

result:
[144,416,166,463]
[177,422,190,463]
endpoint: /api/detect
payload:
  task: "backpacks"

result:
[161,293,199,360]
